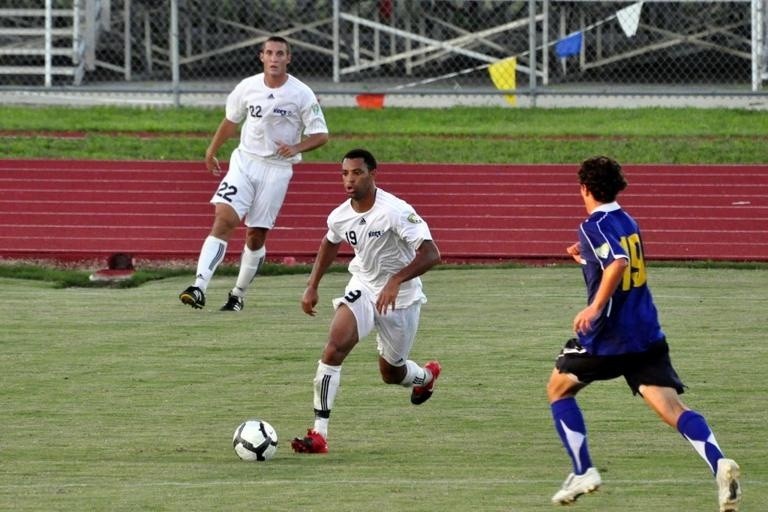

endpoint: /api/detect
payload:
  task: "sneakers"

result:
[289,430,330,454]
[178,284,206,311]
[715,456,743,512]
[549,464,602,505]
[220,291,245,312]
[411,358,440,405]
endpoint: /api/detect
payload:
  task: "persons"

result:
[179,36,329,311]
[290,148,441,453]
[545,156,742,512]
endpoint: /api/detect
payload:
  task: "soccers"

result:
[233,420,278,462]
